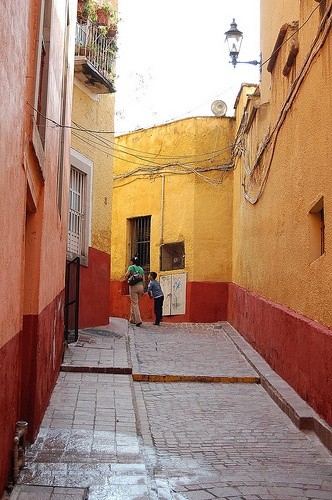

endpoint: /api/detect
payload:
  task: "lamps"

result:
[223,17,262,69]
[211,100,228,118]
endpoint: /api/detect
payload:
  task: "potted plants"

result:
[77,42,117,90]
[77,1,91,25]
[104,16,122,37]
[87,0,117,26]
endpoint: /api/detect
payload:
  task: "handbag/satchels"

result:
[128,272,142,286]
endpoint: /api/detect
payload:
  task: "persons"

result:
[147,271,165,326]
[124,258,147,326]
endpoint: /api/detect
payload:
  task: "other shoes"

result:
[153,320,162,325]
[129,321,136,324]
[136,322,142,327]
